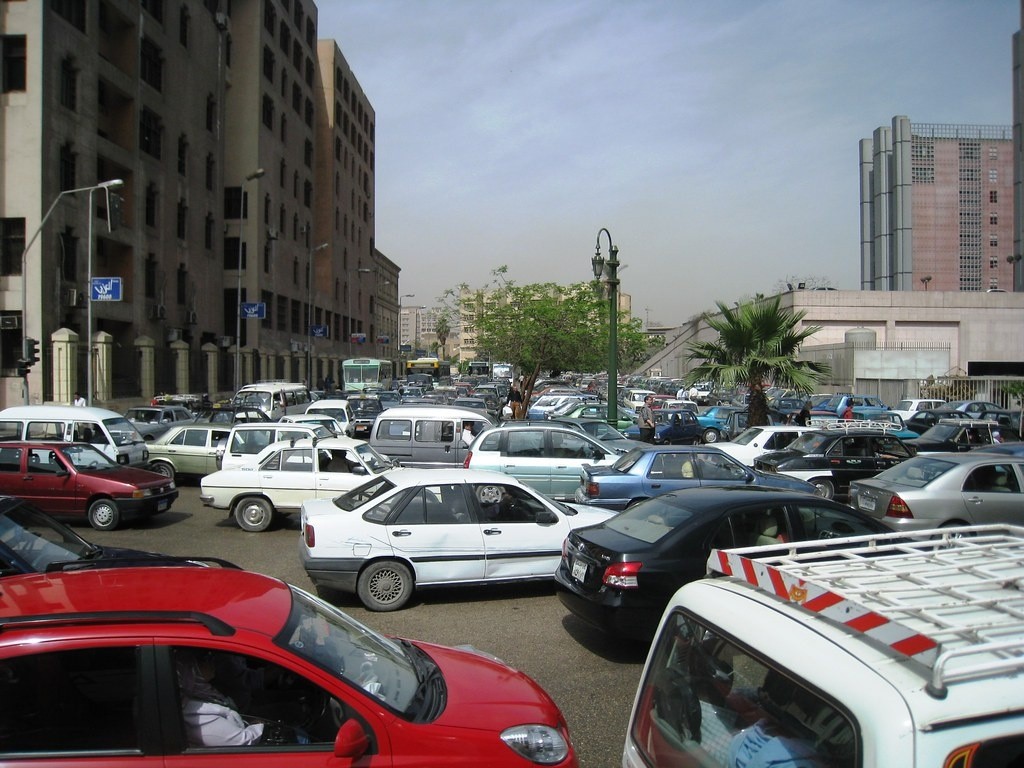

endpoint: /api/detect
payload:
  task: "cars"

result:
[0,553,578,768]
[1,442,182,530]
[0,492,213,575]
[301,466,619,614]
[123,358,1023,532]
[848,452,1023,547]
[553,486,945,643]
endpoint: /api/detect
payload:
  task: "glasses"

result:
[197,650,216,662]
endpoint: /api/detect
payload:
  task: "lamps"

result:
[798,282,805,288]
[787,283,794,291]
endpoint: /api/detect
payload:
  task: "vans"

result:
[624,520,1024,768]
[0,406,155,468]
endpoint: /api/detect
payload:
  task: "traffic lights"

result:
[18,359,31,376]
[25,338,40,366]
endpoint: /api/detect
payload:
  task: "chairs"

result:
[442,487,468,520]
[847,438,867,457]
[907,467,923,481]
[672,414,678,422]
[966,469,1012,494]
[741,512,782,546]
[73,427,93,441]
[664,455,694,477]
[1,451,56,470]
[319,452,332,471]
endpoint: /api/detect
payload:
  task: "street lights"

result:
[306,244,331,390]
[346,267,426,358]
[232,168,265,390]
[17,179,125,404]
[592,228,621,433]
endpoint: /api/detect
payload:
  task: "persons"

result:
[74,392,86,407]
[316,376,337,394]
[842,401,854,422]
[638,396,655,442]
[688,638,828,768]
[327,450,376,473]
[172,647,311,746]
[507,387,523,419]
[689,385,698,402]
[462,422,474,446]
[518,374,525,388]
[800,401,813,426]
[992,431,1005,444]
[676,386,687,400]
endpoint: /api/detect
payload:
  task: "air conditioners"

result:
[67,288,88,307]
[300,224,306,232]
[268,228,279,239]
[219,12,228,27]
[0,315,22,330]
[168,328,182,341]
[221,336,233,348]
[151,304,166,319]
[185,311,198,324]
